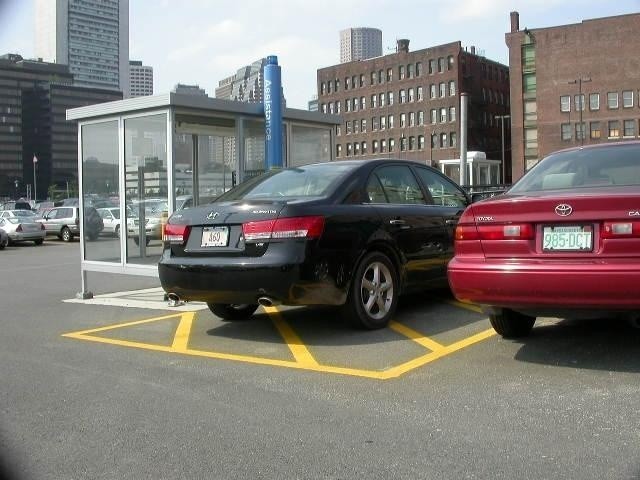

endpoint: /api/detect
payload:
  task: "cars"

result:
[158,158,484,334]
[0,194,214,248]
[449,136,640,341]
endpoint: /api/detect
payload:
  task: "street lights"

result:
[566,74,594,147]
[13,179,19,201]
[33,152,39,200]
[493,113,512,186]
[106,179,112,196]
